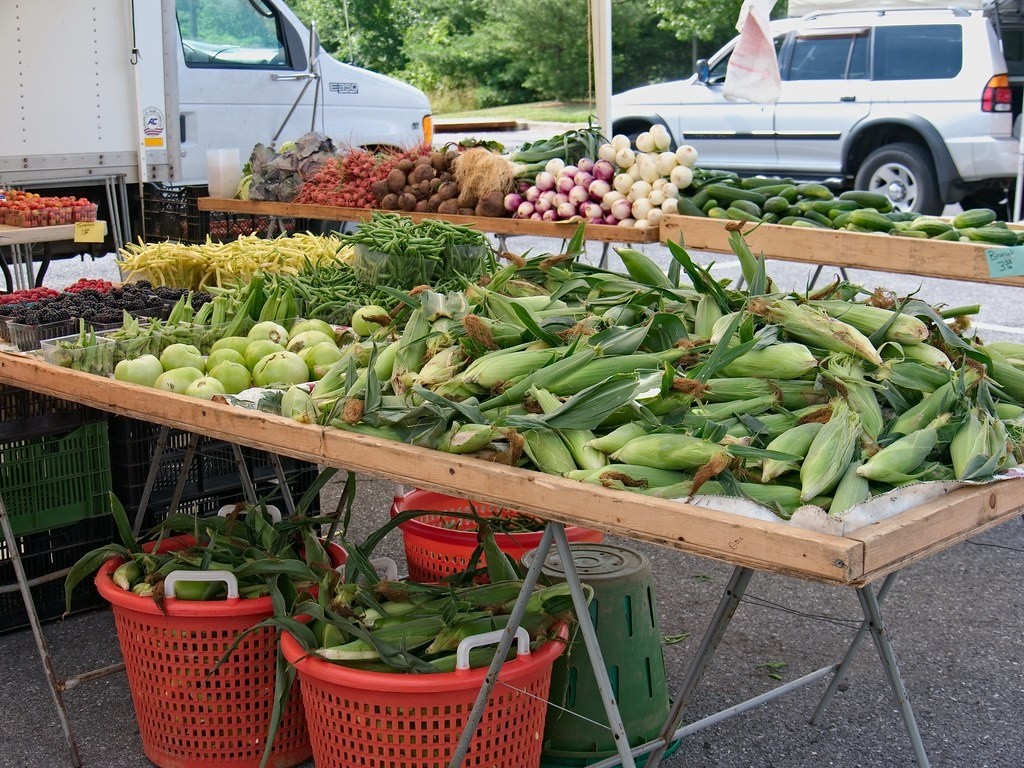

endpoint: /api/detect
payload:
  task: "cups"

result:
[206,148,241,198]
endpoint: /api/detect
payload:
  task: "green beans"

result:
[265,205,489,326]
[114,229,354,289]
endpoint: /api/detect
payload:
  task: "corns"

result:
[281,244,1024,519]
[114,507,575,743]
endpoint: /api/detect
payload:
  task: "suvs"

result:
[608,0,1024,221]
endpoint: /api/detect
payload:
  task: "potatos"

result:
[371,149,503,215]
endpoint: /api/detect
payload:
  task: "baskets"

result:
[281,557,568,768]
[391,483,603,583]
[95,503,351,768]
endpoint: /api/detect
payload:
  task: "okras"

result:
[55,268,295,377]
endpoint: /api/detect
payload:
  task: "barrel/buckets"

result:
[518,541,681,768]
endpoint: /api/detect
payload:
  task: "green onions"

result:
[454,114,610,201]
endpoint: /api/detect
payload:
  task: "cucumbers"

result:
[676,167,1024,247]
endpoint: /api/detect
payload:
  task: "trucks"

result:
[0,0,437,264]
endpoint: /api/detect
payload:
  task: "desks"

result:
[0,220,108,295]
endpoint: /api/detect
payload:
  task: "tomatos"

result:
[0,189,97,227]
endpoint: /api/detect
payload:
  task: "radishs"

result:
[599,125,698,229]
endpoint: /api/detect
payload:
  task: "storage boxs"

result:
[110,413,319,512]
[89,326,163,374]
[179,320,249,354]
[205,148,241,197]
[124,469,322,544]
[142,319,204,358]
[247,315,299,335]
[293,298,304,317]
[3,207,48,229]
[46,207,73,226]
[0,421,114,540]
[353,243,438,291]
[438,243,487,277]
[0,384,109,445]
[71,204,99,224]
[5,317,76,351]
[304,300,350,326]
[0,514,111,638]
[0,315,17,343]
[132,181,305,244]
[84,315,149,332]
[38,333,116,376]
